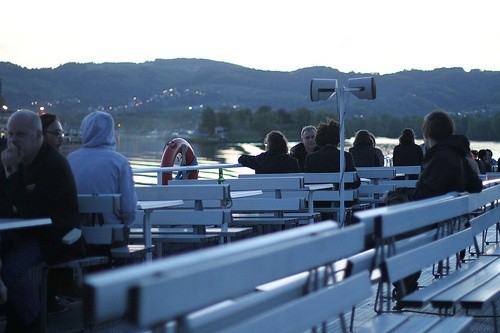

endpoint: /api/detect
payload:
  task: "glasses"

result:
[264,141,268,147]
[43,130,65,138]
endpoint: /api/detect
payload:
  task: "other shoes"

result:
[55,285,82,304]
[47,303,67,316]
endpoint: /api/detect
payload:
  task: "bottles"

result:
[218,168,225,185]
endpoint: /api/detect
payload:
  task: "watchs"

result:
[5,165,17,173]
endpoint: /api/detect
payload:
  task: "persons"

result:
[65,111,136,265]
[0,109,88,333]
[238,111,500,308]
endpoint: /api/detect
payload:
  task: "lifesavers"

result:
[159,137,200,186]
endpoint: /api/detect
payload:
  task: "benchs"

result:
[78,166,500,333]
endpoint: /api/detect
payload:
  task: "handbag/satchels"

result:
[385,193,414,240]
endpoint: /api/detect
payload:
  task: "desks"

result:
[137,199,184,262]
[303,184,333,224]
[222,190,263,243]
